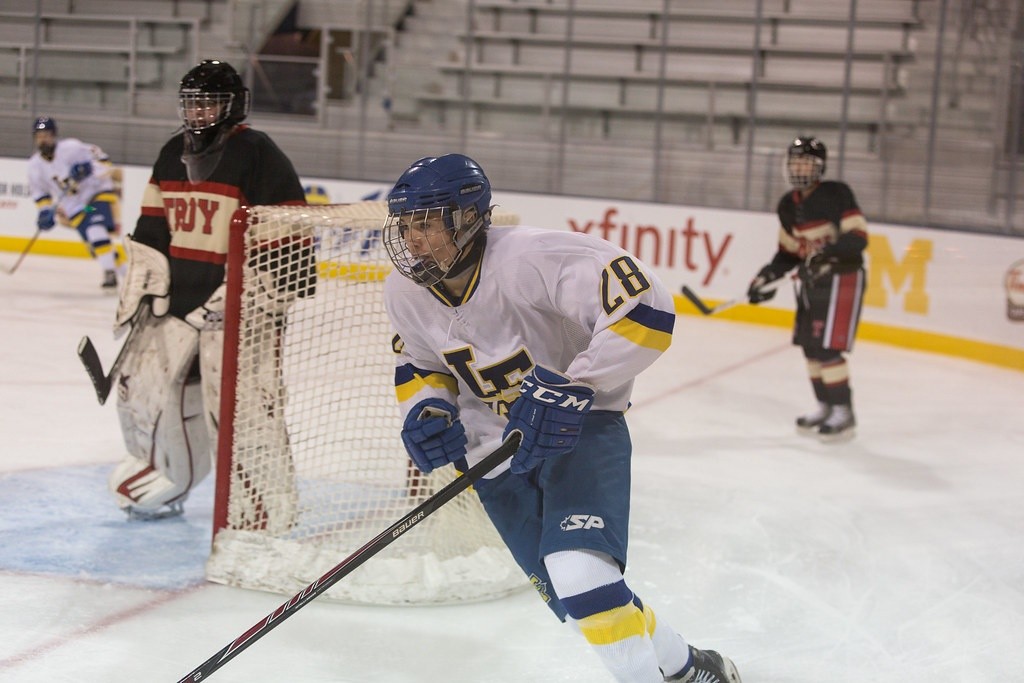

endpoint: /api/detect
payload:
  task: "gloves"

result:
[748,264,779,304]
[400,397,468,473]
[797,249,838,286]
[70,162,91,182]
[501,362,598,474]
[37,210,55,230]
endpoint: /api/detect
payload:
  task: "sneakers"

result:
[658,633,742,683]
[795,389,830,435]
[817,400,856,442]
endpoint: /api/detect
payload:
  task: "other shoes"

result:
[98,270,120,294]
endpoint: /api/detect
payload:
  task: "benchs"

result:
[0,0,1024,250]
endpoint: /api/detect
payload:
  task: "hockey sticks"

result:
[680,270,798,315]
[1,187,67,275]
[78,296,151,406]
[176,434,519,683]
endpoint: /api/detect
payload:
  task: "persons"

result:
[383,154,742,683]
[748,135,869,440]
[27,117,127,287]
[116,59,317,533]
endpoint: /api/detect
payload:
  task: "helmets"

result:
[33,116,57,161]
[382,153,499,286]
[178,59,250,186]
[786,136,827,191]
